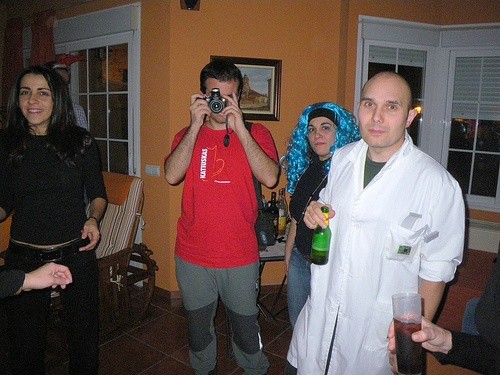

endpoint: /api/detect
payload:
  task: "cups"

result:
[392,292,426,375]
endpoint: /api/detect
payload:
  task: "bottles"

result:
[259,188,289,237]
[311,205,331,265]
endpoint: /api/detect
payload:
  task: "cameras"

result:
[204,88,226,113]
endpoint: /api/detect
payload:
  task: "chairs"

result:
[84,171,159,333]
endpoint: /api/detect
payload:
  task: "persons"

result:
[286,73,465,375]
[164,61,279,375]
[387,242,500,375]
[0,262,73,298]
[0,67,108,375]
[45,61,89,132]
[285,103,362,338]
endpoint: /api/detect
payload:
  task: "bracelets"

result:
[84,216,99,223]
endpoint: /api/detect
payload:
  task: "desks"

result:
[256,218,292,323]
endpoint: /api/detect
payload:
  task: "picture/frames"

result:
[210,55,283,121]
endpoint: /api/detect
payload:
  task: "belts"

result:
[9,236,90,260]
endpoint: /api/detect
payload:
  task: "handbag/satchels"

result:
[255,214,275,246]
